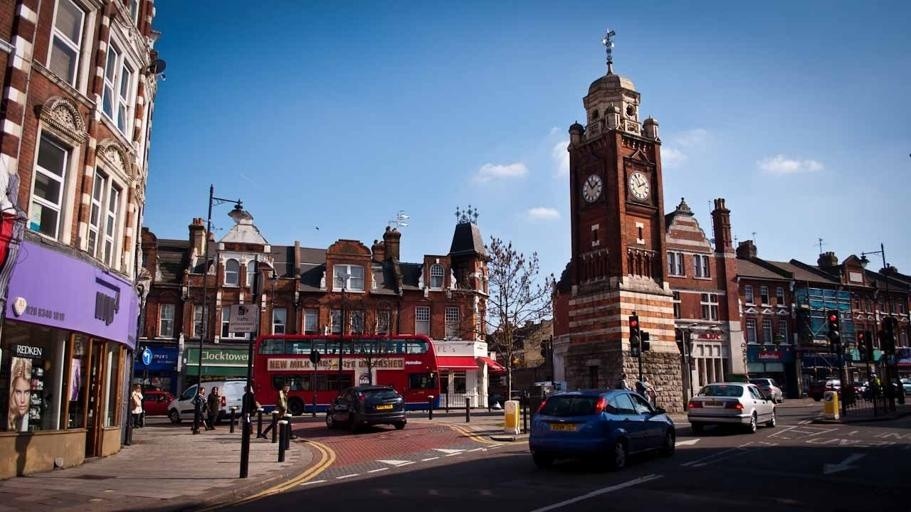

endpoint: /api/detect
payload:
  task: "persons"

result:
[131,384,144,428]
[208,386,221,430]
[830,377,890,410]
[7,355,32,433]
[620,373,658,403]
[241,384,258,434]
[261,383,298,439]
[192,387,210,431]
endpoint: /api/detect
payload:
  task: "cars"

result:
[490,393,505,405]
[688,382,777,433]
[529,388,677,470]
[750,378,785,404]
[808,377,911,402]
[142,388,176,417]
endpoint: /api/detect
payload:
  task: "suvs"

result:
[325,385,407,434]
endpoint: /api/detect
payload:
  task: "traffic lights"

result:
[879,324,892,352]
[829,311,840,340]
[858,333,867,351]
[674,327,683,350]
[540,340,546,357]
[643,330,651,352]
[629,317,640,357]
[689,329,694,343]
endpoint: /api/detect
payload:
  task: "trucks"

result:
[527,381,568,403]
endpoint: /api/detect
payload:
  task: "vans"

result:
[168,379,255,423]
[511,389,524,403]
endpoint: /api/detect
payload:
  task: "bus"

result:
[253,332,442,416]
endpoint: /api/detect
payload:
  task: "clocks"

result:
[628,170,651,203]
[581,173,603,204]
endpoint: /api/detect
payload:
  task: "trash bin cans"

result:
[824,391,839,420]
[504,400,520,435]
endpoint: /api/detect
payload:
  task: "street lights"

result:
[192,182,254,436]
[860,242,906,404]
